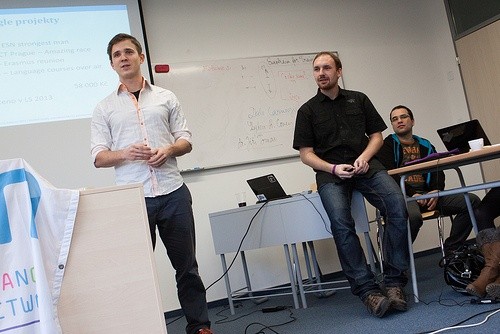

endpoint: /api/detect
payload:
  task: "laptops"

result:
[437,119,491,154]
[247,174,301,204]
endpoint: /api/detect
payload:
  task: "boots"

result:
[485,273,500,298]
[466,228,500,298]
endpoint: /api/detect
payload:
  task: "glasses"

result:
[391,115,410,122]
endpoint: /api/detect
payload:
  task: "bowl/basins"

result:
[468,138,484,150]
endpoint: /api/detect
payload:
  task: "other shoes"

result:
[196,324,214,334]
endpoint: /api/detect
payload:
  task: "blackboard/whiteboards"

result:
[151,50,345,171]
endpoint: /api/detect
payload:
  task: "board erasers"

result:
[155,64,170,73]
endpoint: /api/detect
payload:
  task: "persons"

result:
[91,33,213,334]
[383,106,481,257]
[293,52,408,317]
[465,186,500,301]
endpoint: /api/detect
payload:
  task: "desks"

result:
[387,143,500,302]
[209,188,378,315]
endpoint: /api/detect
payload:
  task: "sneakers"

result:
[362,294,391,318]
[380,286,407,310]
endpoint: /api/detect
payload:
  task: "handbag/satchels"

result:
[438,244,486,288]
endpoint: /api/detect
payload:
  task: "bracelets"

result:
[332,163,336,174]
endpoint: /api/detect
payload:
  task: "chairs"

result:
[381,210,453,265]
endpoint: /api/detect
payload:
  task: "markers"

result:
[346,168,353,171]
